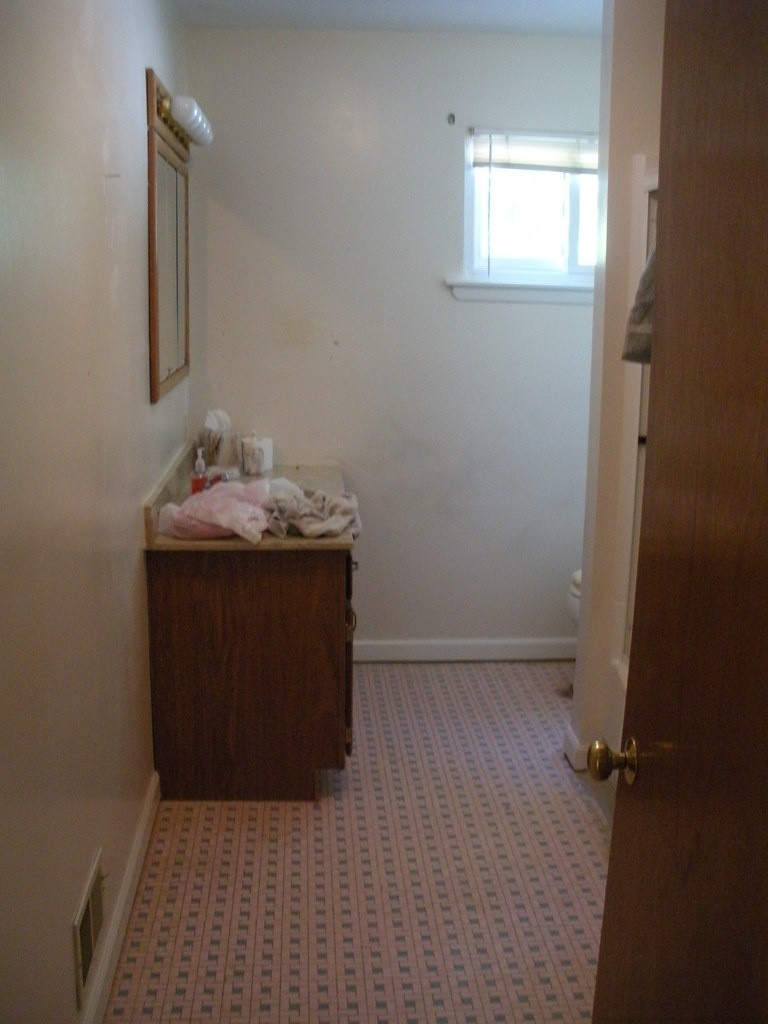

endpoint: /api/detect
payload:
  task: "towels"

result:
[266,490,363,541]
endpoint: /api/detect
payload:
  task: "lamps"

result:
[156,92,217,151]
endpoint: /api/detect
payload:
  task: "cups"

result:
[241,438,273,473]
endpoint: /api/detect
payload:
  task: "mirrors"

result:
[145,131,195,405]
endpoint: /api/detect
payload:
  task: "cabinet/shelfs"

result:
[146,462,361,800]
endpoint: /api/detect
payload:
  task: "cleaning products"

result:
[189,445,210,495]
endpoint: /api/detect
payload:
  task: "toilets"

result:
[566,568,583,626]
